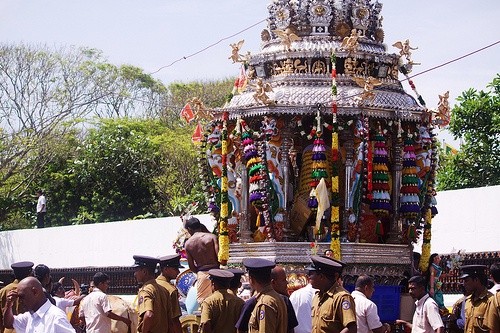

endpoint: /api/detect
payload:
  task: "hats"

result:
[131,255,160,268]
[224,269,244,279]
[458,265,487,278]
[243,258,277,272]
[208,269,234,281]
[305,256,346,274]
[11,262,34,272]
[159,254,185,268]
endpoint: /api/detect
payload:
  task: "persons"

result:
[425,253,444,304]
[0,261,94,333]
[200,268,245,333]
[396,276,444,333]
[432,90,449,117]
[234,267,299,333]
[399,39,418,62]
[130,255,185,333]
[363,77,376,100]
[247,80,266,102]
[228,43,239,62]
[184,218,219,305]
[282,32,291,48]
[458,265,500,333]
[350,275,390,333]
[289,256,357,333]
[79,272,132,333]
[37,189,46,229]
[241,258,288,333]
[347,29,358,53]
